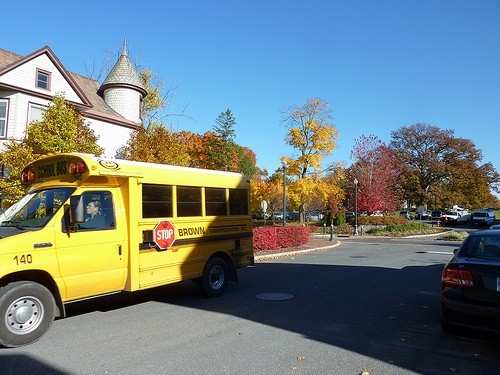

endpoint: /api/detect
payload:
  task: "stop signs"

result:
[153,220,176,250]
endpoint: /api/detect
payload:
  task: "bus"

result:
[0,152,255,349]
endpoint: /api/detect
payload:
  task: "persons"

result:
[78,199,106,228]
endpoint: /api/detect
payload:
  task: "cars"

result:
[254,209,326,222]
[399,211,413,220]
[345,210,383,217]
[440,229,500,335]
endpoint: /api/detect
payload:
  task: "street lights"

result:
[353,178,359,235]
[281,161,287,226]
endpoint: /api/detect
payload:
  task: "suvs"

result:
[415,208,494,228]
